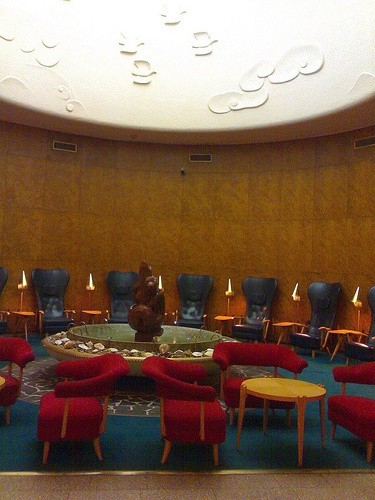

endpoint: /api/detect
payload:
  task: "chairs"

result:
[106,271,138,323]
[0,267,8,336]
[328,361,375,463]
[0,336,34,424]
[32,268,76,337]
[290,280,342,361]
[141,356,226,467]
[171,273,213,330]
[345,286,375,366]
[37,353,130,465]
[213,341,308,427]
[231,276,277,343]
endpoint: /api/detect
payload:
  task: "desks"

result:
[215,315,361,361]
[13,310,102,341]
[235,377,328,467]
